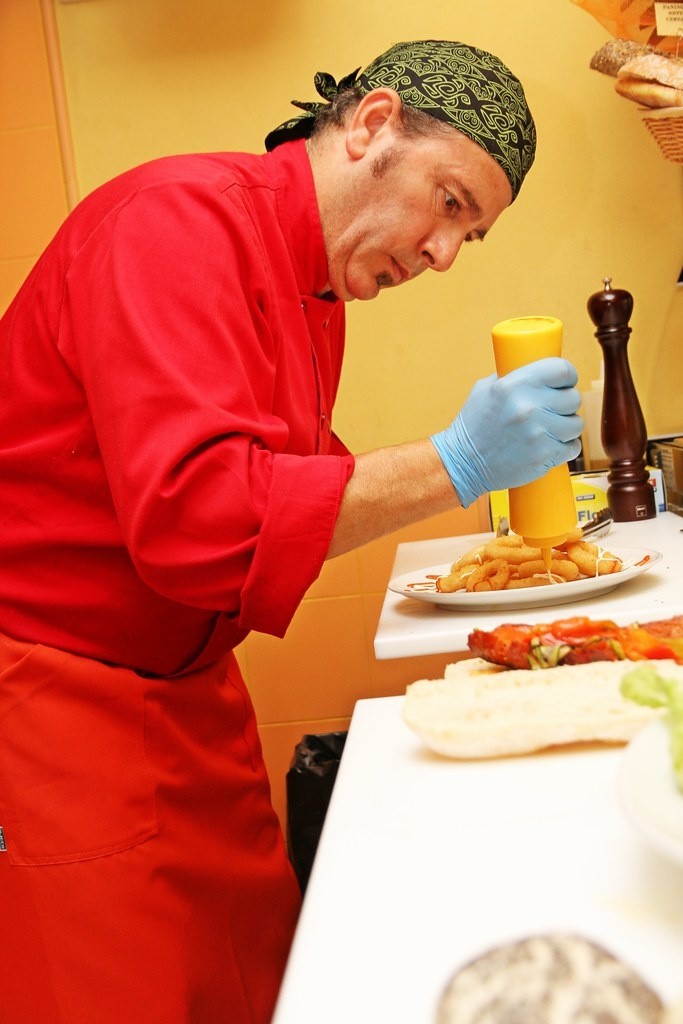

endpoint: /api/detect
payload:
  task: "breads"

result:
[589,38,683,109]
[402,660,681,760]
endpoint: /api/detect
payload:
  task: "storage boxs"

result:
[647,434,683,518]
[488,464,668,532]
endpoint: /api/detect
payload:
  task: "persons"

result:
[0,38,582,1024]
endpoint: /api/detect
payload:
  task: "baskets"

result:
[637,106,683,162]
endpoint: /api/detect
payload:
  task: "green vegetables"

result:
[528,635,626,670]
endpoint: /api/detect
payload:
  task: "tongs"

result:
[496,507,613,543]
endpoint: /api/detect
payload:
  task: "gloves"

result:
[430,356,584,508]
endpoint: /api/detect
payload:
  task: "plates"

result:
[387,546,662,611]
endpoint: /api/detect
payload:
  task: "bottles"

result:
[490,315,578,571]
[582,360,610,469]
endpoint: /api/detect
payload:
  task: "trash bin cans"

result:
[283,730,353,890]
